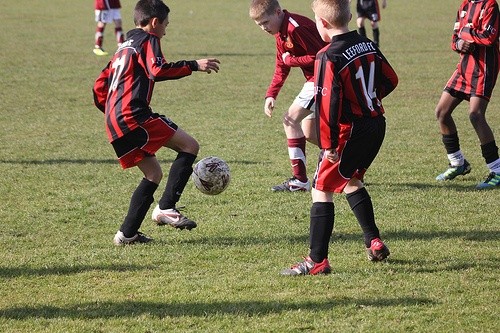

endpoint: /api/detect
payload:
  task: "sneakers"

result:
[477,172,500,189]
[435,158,473,182]
[113,230,155,246]
[368,237,391,262]
[271,178,313,193]
[151,203,198,231]
[92,47,109,56]
[279,257,334,277]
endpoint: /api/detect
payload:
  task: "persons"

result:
[92,0,220,245]
[277,0,399,276]
[435,0,500,190]
[249,0,331,193]
[355,0,386,47]
[93,0,123,56]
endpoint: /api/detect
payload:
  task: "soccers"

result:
[192,157,231,195]
